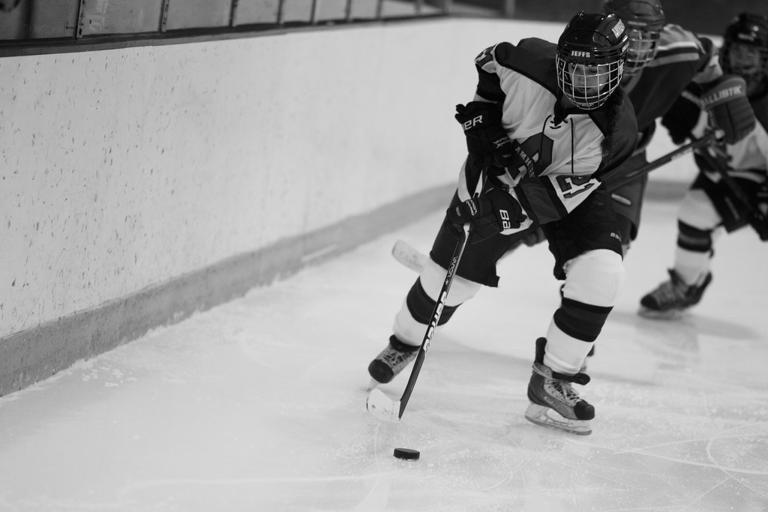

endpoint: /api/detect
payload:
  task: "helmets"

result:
[555,13,630,111]
[605,1,665,78]
[719,13,768,97]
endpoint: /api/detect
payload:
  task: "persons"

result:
[366,0,756,434]
[638,1,767,320]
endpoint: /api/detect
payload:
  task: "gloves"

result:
[447,188,526,244]
[455,101,517,170]
[660,81,703,146]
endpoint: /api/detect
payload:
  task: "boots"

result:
[641,268,712,310]
[369,336,419,383]
[528,335,594,420]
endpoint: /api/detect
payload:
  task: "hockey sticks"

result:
[366,167,488,422]
[392,126,725,273]
